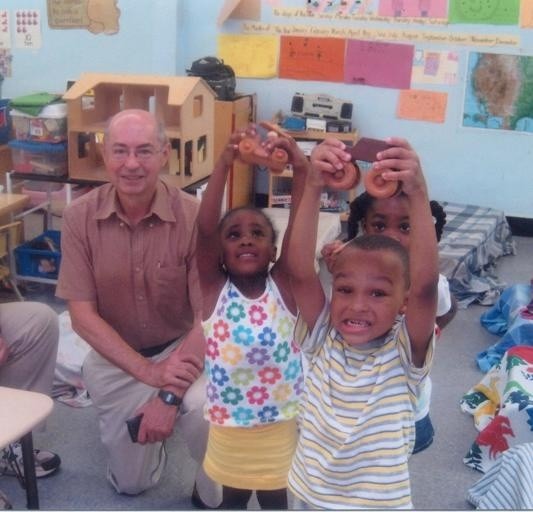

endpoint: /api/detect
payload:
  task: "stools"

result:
[0,386,55,509]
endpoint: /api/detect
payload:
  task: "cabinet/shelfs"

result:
[268,109,359,221]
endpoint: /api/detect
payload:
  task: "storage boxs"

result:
[193,89,257,209]
[12,228,62,279]
[7,140,67,177]
[8,103,67,144]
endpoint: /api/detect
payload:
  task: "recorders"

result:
[291,92,353,121]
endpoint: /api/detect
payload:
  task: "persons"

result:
[194,118,309,511]
[282,130,439,512]
[0,299,62,509]
[54,107,225,509]
[319,178,454,455]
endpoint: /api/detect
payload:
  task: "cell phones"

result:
[125,413,144,442]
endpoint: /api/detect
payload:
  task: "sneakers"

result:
[0,442,61,478]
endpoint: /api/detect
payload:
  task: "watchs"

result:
[157,389,183,409]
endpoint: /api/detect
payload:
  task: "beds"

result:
[431,199,533,510]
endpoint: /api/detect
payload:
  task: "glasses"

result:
[111,146,157,159]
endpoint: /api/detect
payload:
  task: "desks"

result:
[3,169,210,302]
[0,192,31,301]
